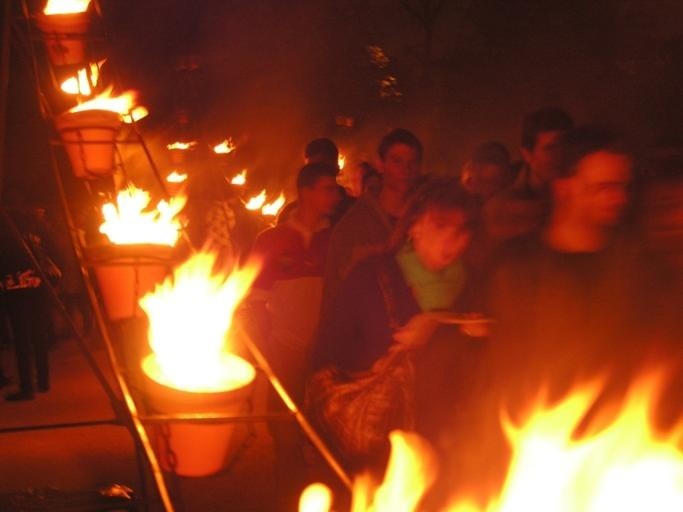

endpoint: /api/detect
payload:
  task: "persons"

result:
[0,201,65,401]
[30,205,98,347]
[233,104,683,512]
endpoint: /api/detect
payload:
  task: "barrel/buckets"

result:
[32,0,92,66]
[51,109,125,179]
[85,241,179,322]
[138,346,256,480]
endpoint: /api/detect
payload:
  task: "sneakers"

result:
[7,391,32,399]
[38,381,47,392]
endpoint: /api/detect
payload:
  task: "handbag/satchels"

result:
[301,365,389,455]
